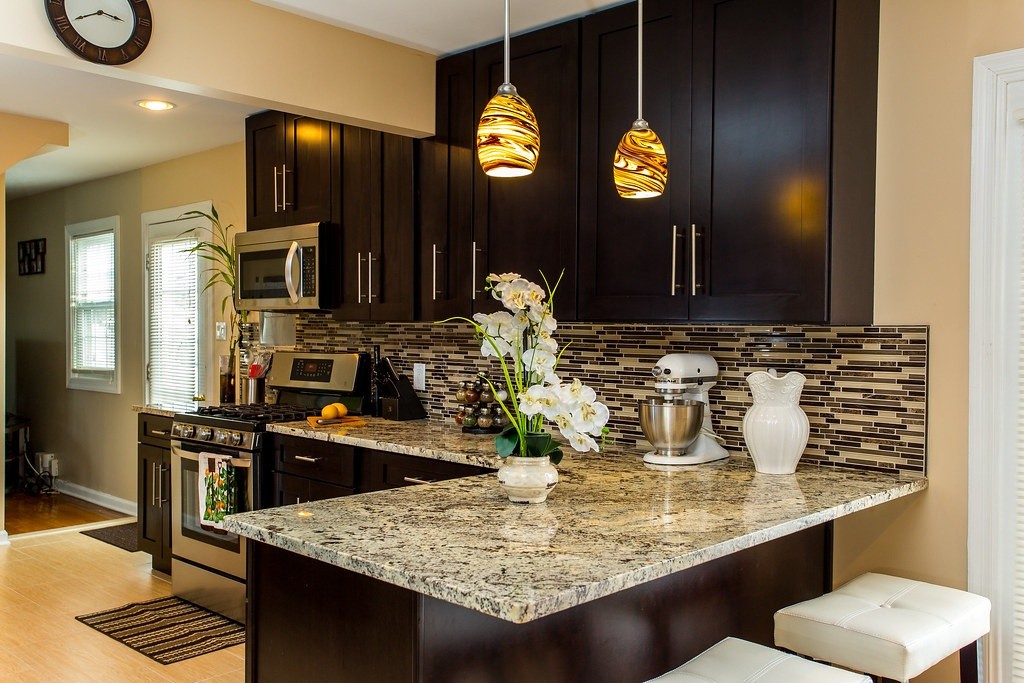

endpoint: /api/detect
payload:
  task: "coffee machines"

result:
[643,353,730,464]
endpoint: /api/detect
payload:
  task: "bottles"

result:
[371,344,382,417]
[456,382,508,427]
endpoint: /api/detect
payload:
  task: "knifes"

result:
[373,357,400,399]
[317,418,362,424]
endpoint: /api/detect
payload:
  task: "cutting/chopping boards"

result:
[306,416,366,430]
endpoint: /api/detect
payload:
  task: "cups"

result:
[242,378,265,406]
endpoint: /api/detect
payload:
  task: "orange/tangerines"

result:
[322,403,348,419]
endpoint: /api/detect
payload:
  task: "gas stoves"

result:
[171,349,372,424]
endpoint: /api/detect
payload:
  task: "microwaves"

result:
[235,221,343,311]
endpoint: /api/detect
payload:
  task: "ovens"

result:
[171,415,275,625]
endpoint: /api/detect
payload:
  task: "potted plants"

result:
[163,202,242,404]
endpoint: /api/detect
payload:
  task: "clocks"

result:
[43,0,153,66]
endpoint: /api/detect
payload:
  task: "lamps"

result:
[476,0,539,178]
[614,0,667,198]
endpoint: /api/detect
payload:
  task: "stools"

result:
[773,572,992,683]
[643,637,875,683]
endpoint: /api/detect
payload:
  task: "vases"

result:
[497,455,560,504]
[742,371,810,475]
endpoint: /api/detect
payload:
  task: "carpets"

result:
[79,522,141,552]
[74,595,245,665]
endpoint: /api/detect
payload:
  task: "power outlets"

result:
[413,363,425,390]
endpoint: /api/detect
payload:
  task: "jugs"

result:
[742,371,811,474]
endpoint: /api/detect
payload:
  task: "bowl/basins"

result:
[637,400,706,456]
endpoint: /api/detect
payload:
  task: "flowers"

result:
[435,271,619,465]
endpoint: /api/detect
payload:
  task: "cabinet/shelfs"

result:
[5,412,29,494]
[245,522,834,683]
[416,17,587,322]
[138,413,173,576]
[330,121,416,322]
[271,434,499,508]
[588,0,880,325]
[245,111,331,315]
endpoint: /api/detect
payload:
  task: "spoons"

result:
[244,349,254,379]
